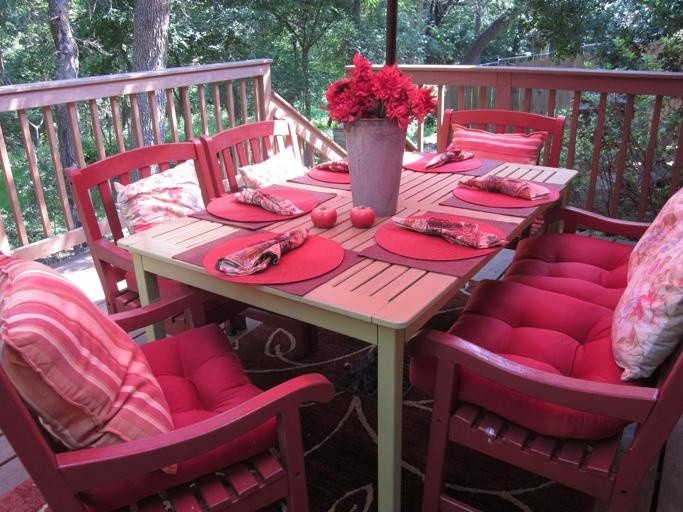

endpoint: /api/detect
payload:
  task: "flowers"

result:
[321,54,439,128]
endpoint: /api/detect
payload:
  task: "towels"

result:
[318,156,349,176]
[230,189,302,220]
[459,171,547,204]
[214,229,312,276]
[424,146,471,171]
[389,210,506,253]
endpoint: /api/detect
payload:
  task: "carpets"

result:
[0,323,591,512]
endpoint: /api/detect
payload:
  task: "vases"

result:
[340,112,406,219]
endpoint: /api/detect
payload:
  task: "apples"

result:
[351,206,375,228]
[310,205,336,229]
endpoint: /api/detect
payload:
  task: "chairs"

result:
[204,116,311,191]
[62,136,246,340]
[505,189,682,293]
[419,218,681,511]
[436,108,564,169]
[0,244,341,510]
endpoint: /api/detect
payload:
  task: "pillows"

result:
[0,252,176,452]
[610,241,679,381]
[623,189,682,284]
[228,147,307,192]
[110,158,204,234]
[443,124,550,169]
